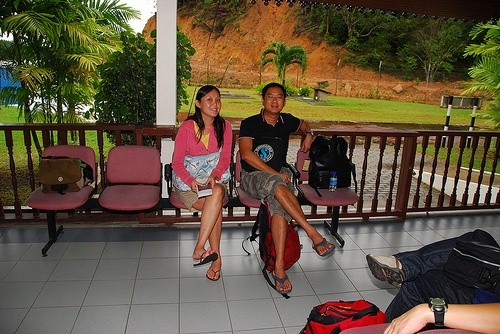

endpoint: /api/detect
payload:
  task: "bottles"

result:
[328,171,337,192]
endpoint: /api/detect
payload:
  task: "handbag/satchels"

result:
[172,151,232,191]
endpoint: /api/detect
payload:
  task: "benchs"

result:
[25,144,359,259]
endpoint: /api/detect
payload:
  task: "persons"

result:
[366,229,500,323]
[172,85,232,282]
[239,82,334,294]
[340,297,500,334]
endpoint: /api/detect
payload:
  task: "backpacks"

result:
[308,136,352,189]
[249,199,301,272]
[299,298,386,334]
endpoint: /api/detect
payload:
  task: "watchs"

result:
[306,131,314,136]
[428,298,448,327]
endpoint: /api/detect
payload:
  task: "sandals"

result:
[366,254,405,290]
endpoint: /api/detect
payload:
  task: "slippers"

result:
[192,251,218,266]
[205,254,221,281]
[312,238,335,260]
[272,270,292,294]
